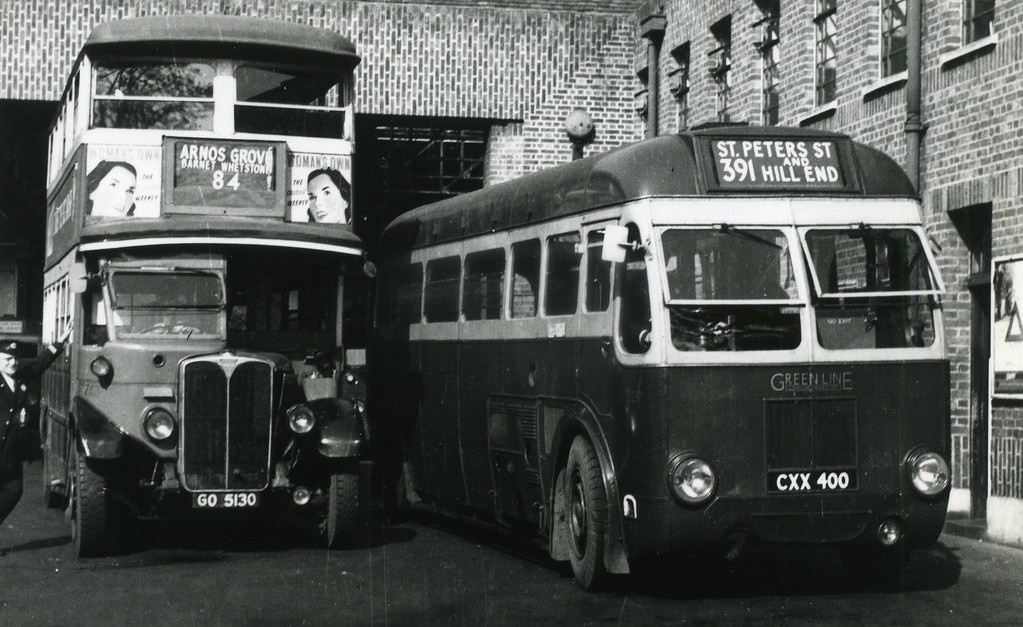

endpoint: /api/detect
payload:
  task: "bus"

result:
[377,126,951,592]
[41,15,367,559]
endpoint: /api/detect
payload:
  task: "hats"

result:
[0,340,23,354]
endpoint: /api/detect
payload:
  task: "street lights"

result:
[565,111,592,162]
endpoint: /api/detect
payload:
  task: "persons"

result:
[305,167,351,224]
[86,159,136,217]
[0,317,74,526]
[624,320,691,352]
[148,298,200,334]
